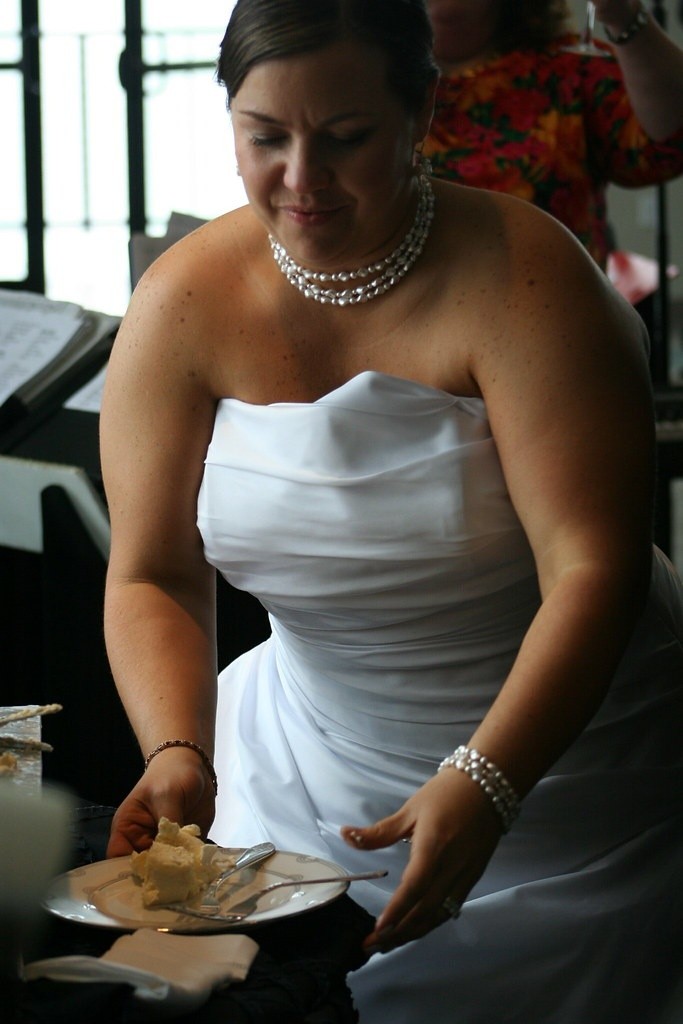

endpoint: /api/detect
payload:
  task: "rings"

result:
[440,898,461,920]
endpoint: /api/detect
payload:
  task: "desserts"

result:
[130,815,239,908]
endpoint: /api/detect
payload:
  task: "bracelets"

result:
[143,740,218,797]
[436,743,523,835]
[603,3,651,46]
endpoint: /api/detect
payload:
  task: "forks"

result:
[163,842,275,914]
[163,869,389,922]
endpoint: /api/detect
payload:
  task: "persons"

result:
[419,0,682,287]
[97,0,682,1023]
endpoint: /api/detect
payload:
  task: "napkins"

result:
[15,927,261,1007]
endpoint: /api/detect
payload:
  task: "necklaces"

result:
[263,166,435,308]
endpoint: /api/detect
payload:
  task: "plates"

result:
[42,846,349,929]
